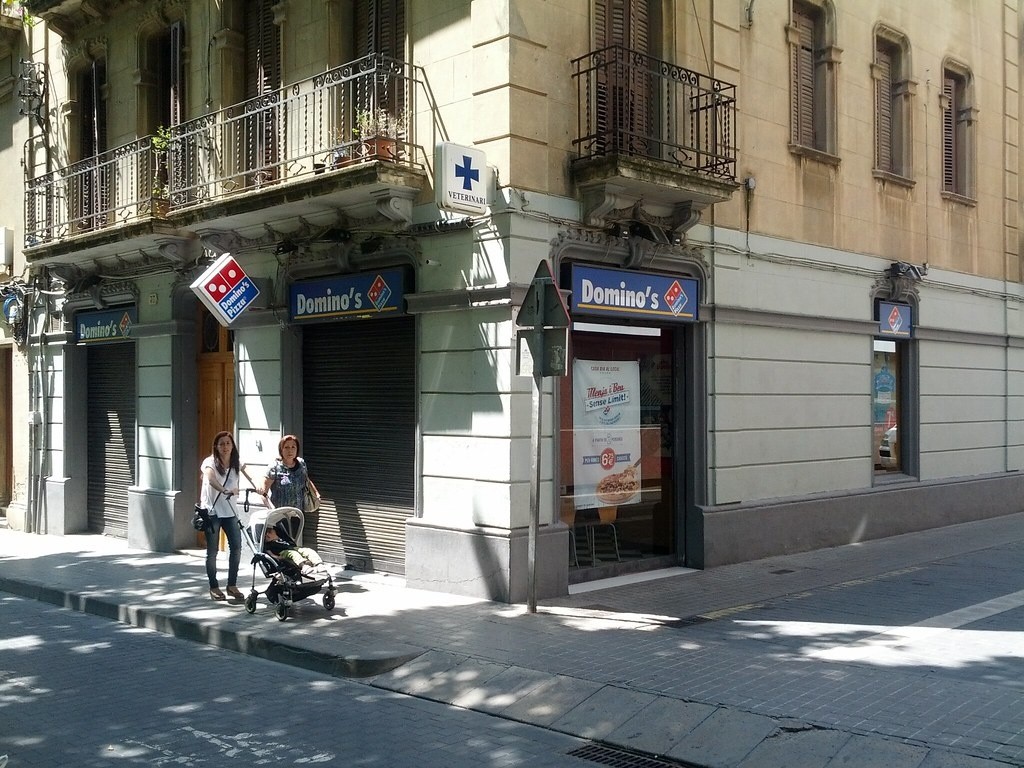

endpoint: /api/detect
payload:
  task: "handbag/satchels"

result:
[190,503,211,529]
[301,464,320,513]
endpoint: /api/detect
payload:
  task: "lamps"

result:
[318,229,351,242]
[72,275,99,294]
[610,221,681,244]
[360,235,386,256]
[883,260,930,282]
[273,239,299,255]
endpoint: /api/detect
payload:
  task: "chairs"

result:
[559,495,621,569]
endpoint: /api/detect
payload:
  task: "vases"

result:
[335,157,350,169]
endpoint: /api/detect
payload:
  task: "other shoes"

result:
[301,566,313,575]
[316,565,327,573]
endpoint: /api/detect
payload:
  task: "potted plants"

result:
[352,105,412,162]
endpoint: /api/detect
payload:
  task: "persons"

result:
[256,434,322,514]
[190,430,245,601]
[262,527,327,575]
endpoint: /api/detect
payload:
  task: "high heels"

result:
[210,590,225,600]
[227,586,244,598]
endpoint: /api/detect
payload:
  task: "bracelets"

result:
[223,490,228,493]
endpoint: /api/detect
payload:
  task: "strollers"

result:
[227,486,338,623]
